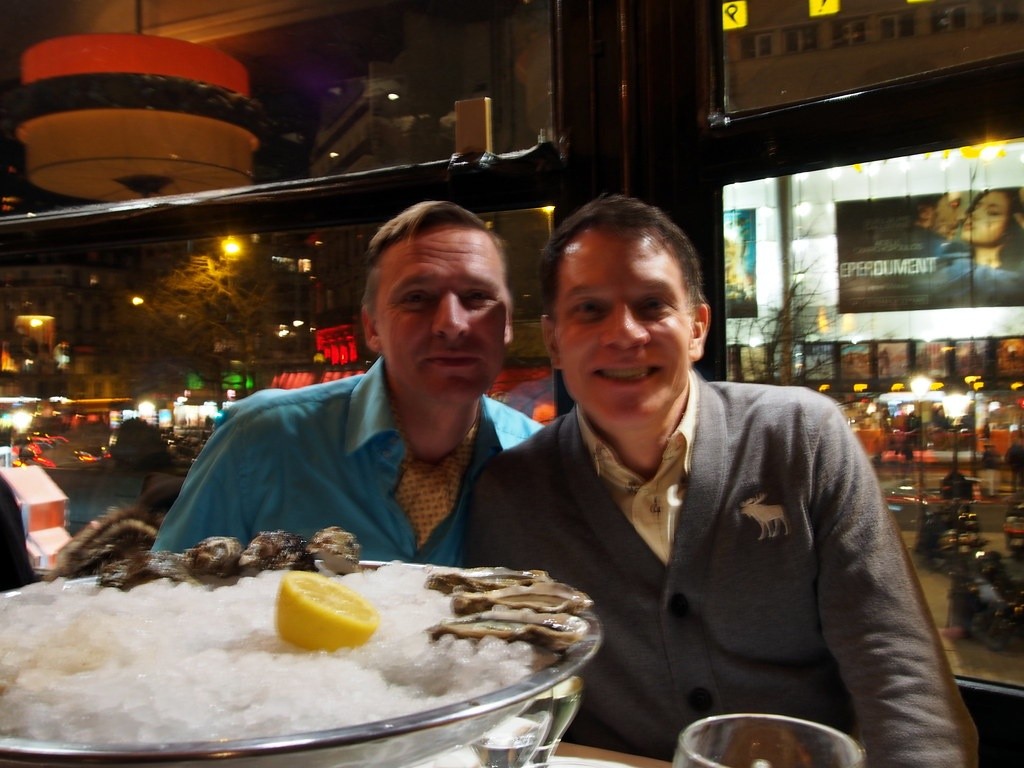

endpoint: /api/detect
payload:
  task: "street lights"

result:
[221,235,243,291]
[910,376,931,501]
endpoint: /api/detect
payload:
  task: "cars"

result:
[883,489,935,529]
[10,432,97,467]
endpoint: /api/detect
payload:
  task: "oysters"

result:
[425,564,594,651]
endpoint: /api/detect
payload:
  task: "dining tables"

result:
[553,741,673,768]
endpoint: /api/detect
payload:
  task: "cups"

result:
[528,676,584,768]
[672,713,866,768]
[471,687,553,768]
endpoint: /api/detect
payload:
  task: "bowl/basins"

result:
[0,563,601,768]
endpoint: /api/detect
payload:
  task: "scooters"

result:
[1002,504,1024,554]
[947,550,1024,653]
[914,501,1000,584]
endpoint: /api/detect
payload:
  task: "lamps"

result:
[14,0,267,202]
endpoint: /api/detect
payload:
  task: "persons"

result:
[982,444,1001,496]
[899,188,1024,306]
[468,196,979,768]
[149,202,548,584]
[1006,440,1024,492]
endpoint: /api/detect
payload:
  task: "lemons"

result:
[274,571,378,651]
